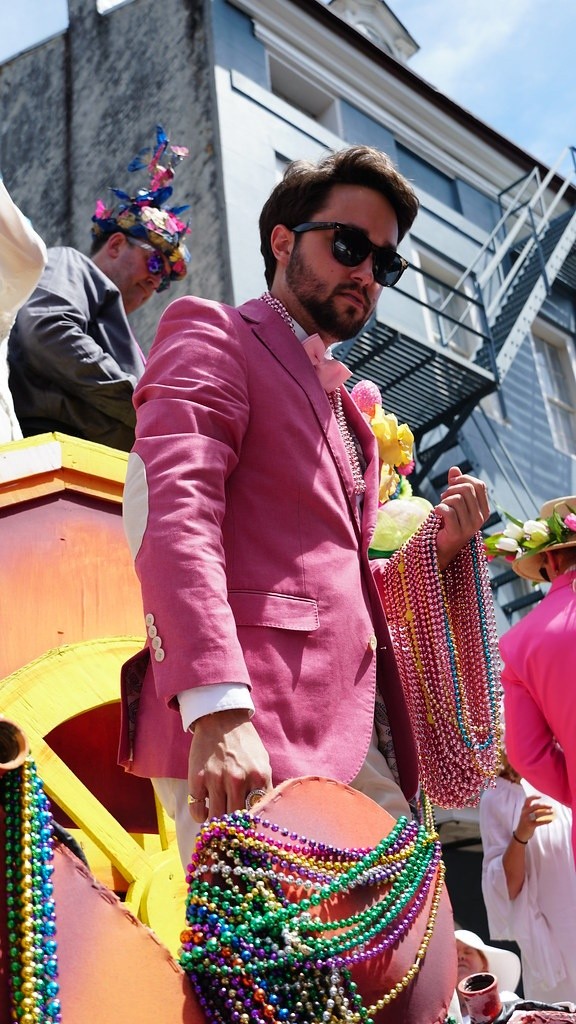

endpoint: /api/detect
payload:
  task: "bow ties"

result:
[300,333,353,394]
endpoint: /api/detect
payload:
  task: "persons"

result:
[477,504,576,1010]
[448,928,521,1024]
[8,198,188,455]
[116,145,416,883]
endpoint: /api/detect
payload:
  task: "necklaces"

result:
[381,509,504,810]
[0,753,61,1024]
[255,293,366,494]
[180,817,446,1024]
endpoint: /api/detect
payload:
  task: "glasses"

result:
[292,222,409,288]
[127,236,171,294]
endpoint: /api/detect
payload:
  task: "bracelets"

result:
[460,1002,467,1007]
[512,831,528,844]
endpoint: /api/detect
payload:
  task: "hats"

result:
[483,495,576,582]
[92,123,189,280]
[447,930,522,1021]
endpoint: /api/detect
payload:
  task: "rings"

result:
[528,813,536,821]
[246,789,267,810]
[533,805,537,811]
[188,795,200,805]
